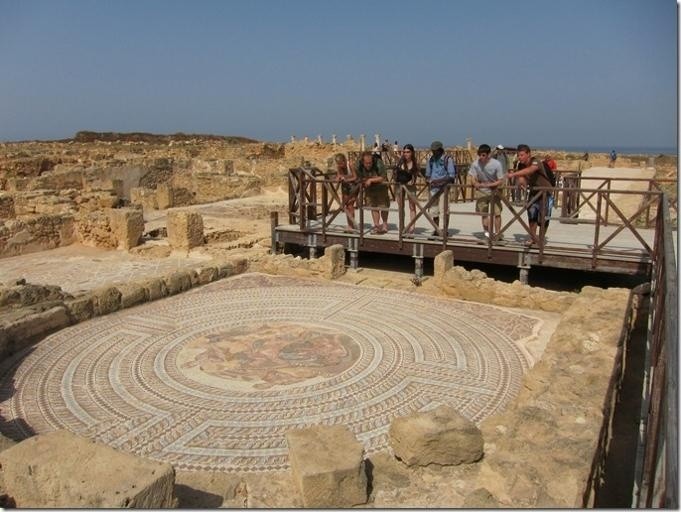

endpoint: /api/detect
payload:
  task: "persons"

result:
[372,138,400,163]
[354,150,390,235]
[466,144,505,242]
[424,141,456,242]
[607,149,617,169]
[393,144,418,239]
[334,152,359,233]
[501,145,554,249]
[490,144,521,205]
[583,153,588,161]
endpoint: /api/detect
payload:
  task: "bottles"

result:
[496,145,504,149]
[431,141,442,151]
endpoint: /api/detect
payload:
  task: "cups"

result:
[478,154,486,157]
[403,151,410,153]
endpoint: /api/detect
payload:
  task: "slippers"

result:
[531,157,557,188]
[396,166,411,183]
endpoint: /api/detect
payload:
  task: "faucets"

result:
[371,225,388,234]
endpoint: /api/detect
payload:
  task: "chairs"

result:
[428,232,448,241]
[478,237,505,246]
